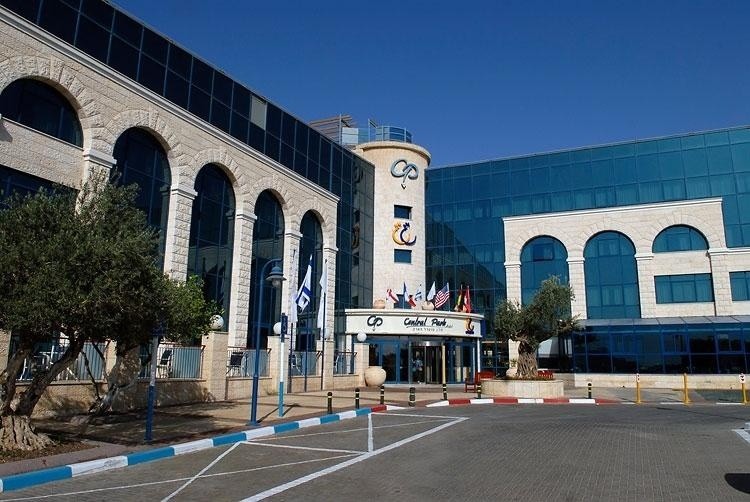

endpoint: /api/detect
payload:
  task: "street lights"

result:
[267,320,288,394]
[246,258,287,427]
[202,314,228,404]
[354,330,370,383]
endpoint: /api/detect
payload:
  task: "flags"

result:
[403,283,416,307]
[427,281,436,301]
[386,289,400,304]
[454,287,464,312]
[434,282,450,309]
[463,286,472,313]
[294,254,313,311]
[415,287,422,302]
